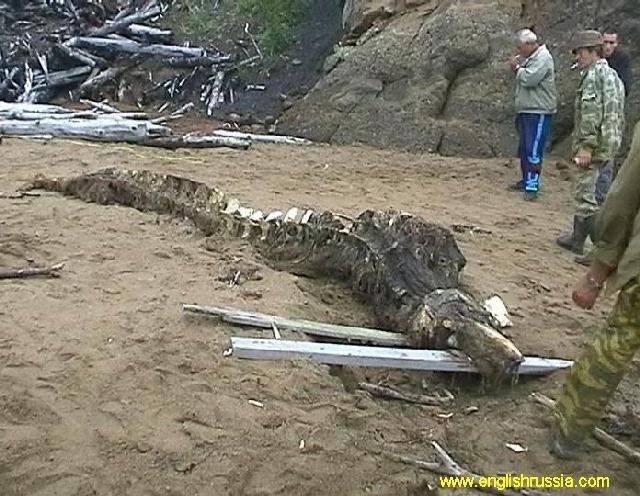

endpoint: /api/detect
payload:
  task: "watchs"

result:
[513,65,522,71]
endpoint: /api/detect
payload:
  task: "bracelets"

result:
[586,273,603,293]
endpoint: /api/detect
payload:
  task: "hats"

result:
[572,29,604,55]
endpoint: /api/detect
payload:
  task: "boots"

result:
[574,214,597,266]
[557,214,588,256]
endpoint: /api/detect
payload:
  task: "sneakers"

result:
[551,417,581,461]
[523,192,537,202]
[507,180,525,192]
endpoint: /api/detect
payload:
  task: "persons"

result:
[556,30,624,267]
[515,54,520,58]
[540,121,640,459]
[505,28,558,201]
[595,28,632,207]
